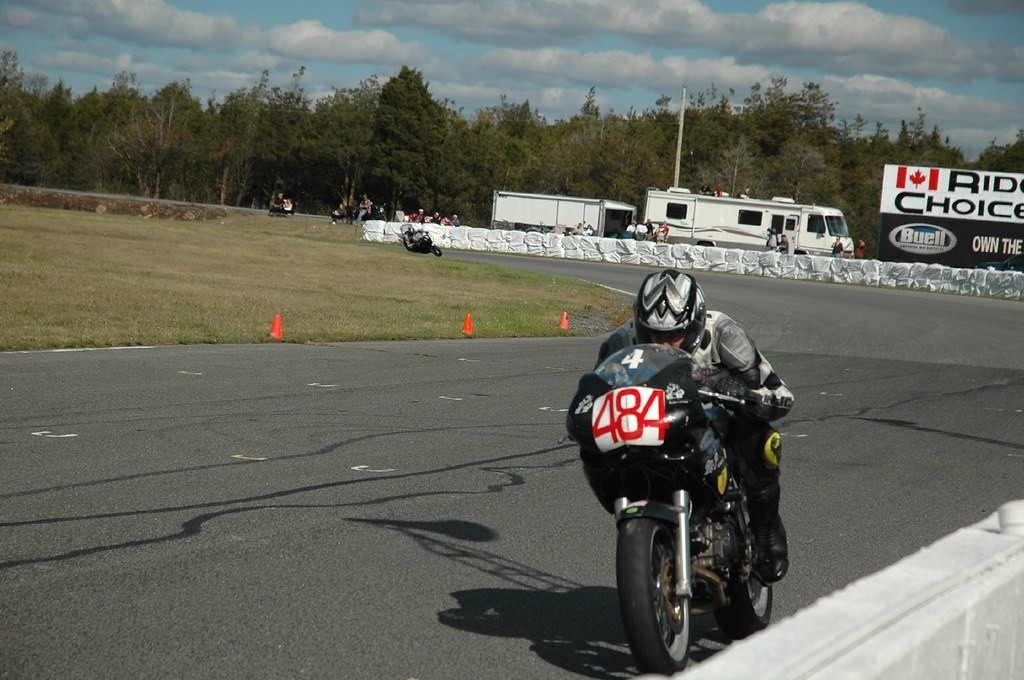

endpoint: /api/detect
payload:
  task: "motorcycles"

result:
[558,343,773,679]
[400,224,442,258]
[363,203,388,221]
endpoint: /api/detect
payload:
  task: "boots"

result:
[743,481,789,584]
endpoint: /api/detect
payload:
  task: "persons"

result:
[593,269,795,583]
[768,226,789,254]
[831,236,844,258]
[359,194,372,220]
[443,214,451,226]
[330,204,345,225]
[267,192,294,217]
[414,208,425,223]
[574,223,593,236]
[451,215,460,226]
[854,239,865,259]
[626,218,669,243]
[430,212,441,225]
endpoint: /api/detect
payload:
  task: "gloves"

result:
[712,376,746,412]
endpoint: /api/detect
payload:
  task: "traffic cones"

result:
[269,314,283,339]
[559,311,568,331]
[462,313,474,334]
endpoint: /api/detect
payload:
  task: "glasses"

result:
[644,327,688,344]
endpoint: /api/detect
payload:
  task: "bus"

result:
[643,186,854,262]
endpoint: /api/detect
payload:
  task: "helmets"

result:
[633,269,712,356]
